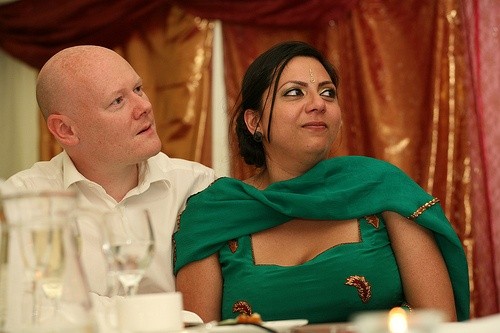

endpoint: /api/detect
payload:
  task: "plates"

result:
[204,318,308,332]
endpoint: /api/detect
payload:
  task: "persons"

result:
[3,45,232,331]
[171,40,469,333]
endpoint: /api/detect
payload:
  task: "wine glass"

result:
[19,204,88,333]
[102,207,158,296]
